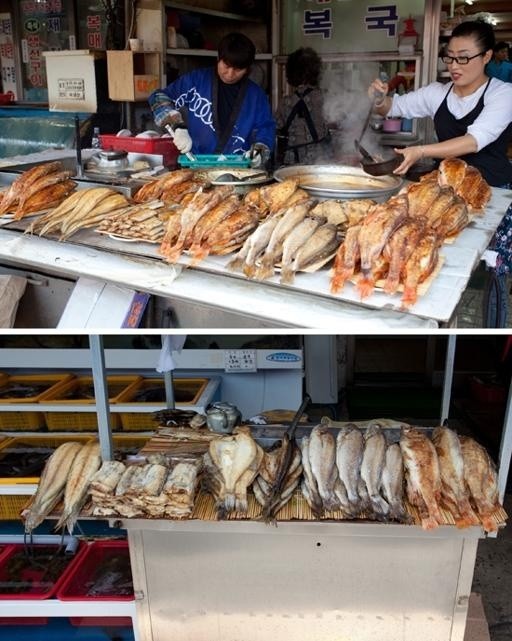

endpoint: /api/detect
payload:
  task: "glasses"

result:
[440,52,486,65]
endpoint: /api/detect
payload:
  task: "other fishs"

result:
[0,156,492,308]
[202,422,509,536]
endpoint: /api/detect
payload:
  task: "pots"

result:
[275,163,404,203]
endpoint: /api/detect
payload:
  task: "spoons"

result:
[215,173,269,181]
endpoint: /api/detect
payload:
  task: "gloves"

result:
[172,127,192,154]
[245,147,263,168]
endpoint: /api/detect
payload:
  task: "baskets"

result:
[99,134,178,166]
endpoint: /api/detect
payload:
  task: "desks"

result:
[2,145,511,328]
[110,423,508,639]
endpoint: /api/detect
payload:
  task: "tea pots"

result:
[205,398,243,436]
[369,114,382,132]
[91,146,128,168]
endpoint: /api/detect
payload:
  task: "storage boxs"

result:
[51,273,152,330]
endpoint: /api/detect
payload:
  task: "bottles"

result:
[89,126,101,149]
[402,118,412,131]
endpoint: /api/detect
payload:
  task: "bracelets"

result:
[418,145,425,162]
[376,98,386,108]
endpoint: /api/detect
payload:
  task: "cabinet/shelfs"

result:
[148,0,278,155]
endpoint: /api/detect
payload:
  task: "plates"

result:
[114,129,170,139]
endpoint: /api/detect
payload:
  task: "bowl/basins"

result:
[1,94,12,105]
[360,146,401,177]
[382,119,402,131]
[205,169,274,186]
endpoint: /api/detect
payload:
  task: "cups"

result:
[129,38,140,52]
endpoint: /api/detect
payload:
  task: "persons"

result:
[368,19,512,326]
[277,44,342,167]
[485,37,512,83]
[147,30,275,170]
